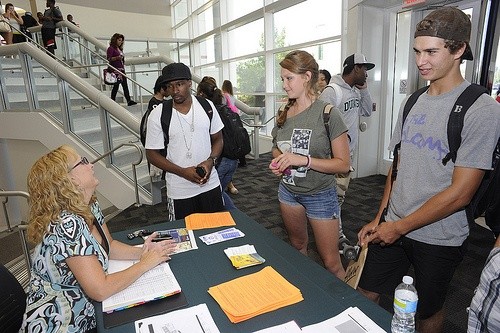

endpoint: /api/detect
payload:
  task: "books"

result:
[102,242,181,314]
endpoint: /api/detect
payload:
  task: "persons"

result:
[269,50,350,281]
[147,75,171,111]
[314,70,331,94]
[107,33,137,106]
[91,46,103,64]
[19,145,177,333]
[145,63,227,222]
[196,76,238,208]
[318,53,375,254]
[467,234,500,333]
[222,80,248,168]
[357,7,500,333]
[0,0,80,59]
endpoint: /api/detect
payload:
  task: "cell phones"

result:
[196,166,206,177]
[273,163,291,175]
[152,233,173,240]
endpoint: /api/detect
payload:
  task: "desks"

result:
[95,205,418,333]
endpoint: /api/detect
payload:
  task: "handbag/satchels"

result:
[104,68,121,85]
[0,14,12,33]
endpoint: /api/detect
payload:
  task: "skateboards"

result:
[337,234,369,289]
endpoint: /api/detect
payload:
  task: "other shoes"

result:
[128,101,137,106]
[228,186,239,195]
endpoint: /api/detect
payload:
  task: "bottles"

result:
[391,276,419,333]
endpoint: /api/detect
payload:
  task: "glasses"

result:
[68,157,89,171]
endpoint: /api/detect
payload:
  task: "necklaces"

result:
[172,97,195,159]
[293,94,312,140]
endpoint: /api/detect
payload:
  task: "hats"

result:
[159,63,191,87]
[414,6,474,61]
[342,53,375,70]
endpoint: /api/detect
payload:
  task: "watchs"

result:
[208,156,218,165]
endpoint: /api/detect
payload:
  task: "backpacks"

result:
[140,96,213,153]
[391,86,500,222]
[214,103,251,167]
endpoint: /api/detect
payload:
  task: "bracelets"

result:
[305,156,311,167]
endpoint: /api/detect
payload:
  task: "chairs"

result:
[0,261,27,333]
